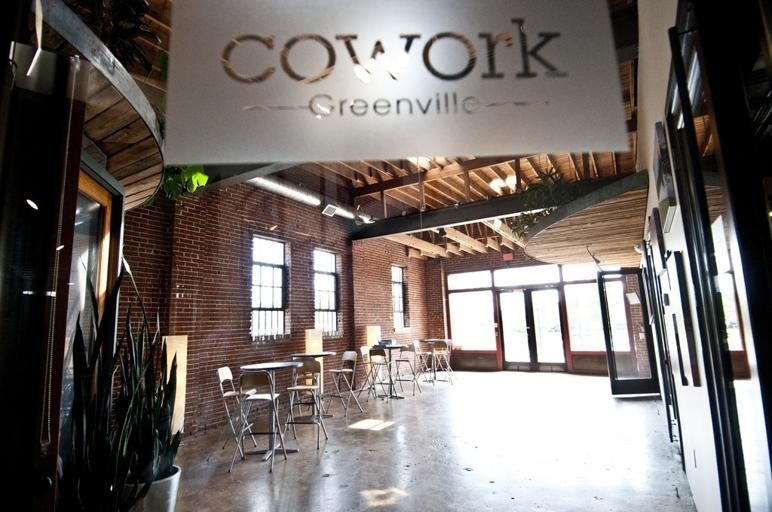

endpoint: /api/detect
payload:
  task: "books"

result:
[381,338,397,345]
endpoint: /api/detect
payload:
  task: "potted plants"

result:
[110,254,183,512]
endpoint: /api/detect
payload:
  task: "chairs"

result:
[216,338,456,473]
